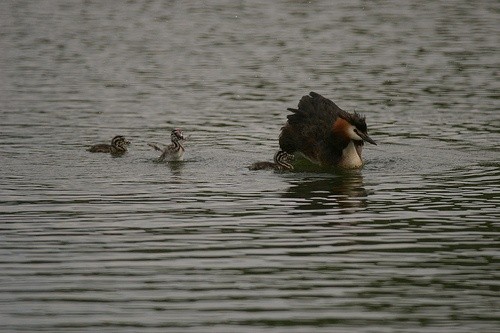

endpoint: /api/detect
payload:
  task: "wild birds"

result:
[249,150,296,174]
[88,135,131,155]
[147,128,192,163]
[277,90,378,171]
